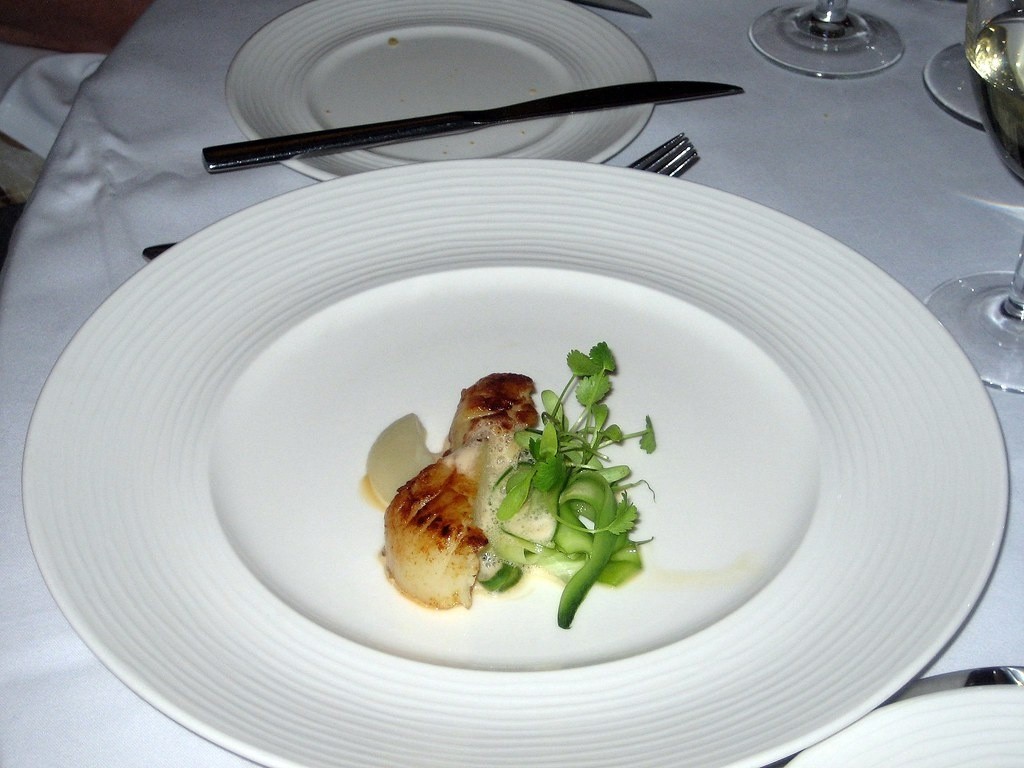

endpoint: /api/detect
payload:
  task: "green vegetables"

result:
[478,340,659,629]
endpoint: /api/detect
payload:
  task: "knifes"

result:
[202,80,744,173]
[759,665,1023,764]
[569,0,652,20]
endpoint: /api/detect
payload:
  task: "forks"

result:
[140,131,701,269]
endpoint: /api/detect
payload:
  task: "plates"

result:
[20,157,1009,767]
[222,1,660,182]
[779,678,1023,768]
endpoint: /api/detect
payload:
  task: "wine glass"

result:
[748,0,905,77]
[921,0,1011,133]
[961,0,1024,395]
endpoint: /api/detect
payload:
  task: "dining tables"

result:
[0,0,1024,768]
